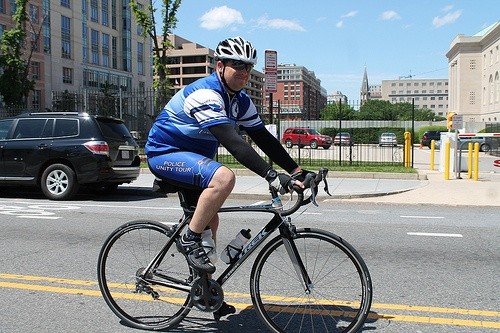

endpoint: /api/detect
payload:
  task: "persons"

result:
[145,37,317,322]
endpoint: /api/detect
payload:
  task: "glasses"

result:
[224,60,255,72]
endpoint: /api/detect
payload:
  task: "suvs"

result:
[282,127,333,150]
[0,111,142,201]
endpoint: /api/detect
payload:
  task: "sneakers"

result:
[176,234,216,274]
[213,302,236,316]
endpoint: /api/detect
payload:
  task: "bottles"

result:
[200,225,218,266]
[220,226,251,264]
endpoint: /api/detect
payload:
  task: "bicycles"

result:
[96,167,375,333]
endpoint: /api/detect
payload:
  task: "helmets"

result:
[214,36,257,64]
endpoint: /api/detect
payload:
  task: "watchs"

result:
[265,169,279,182]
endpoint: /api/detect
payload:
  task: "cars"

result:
[434,136,497,152]
[379,133,398,147]
[421,130,448,149]
[333,132,351,146]
